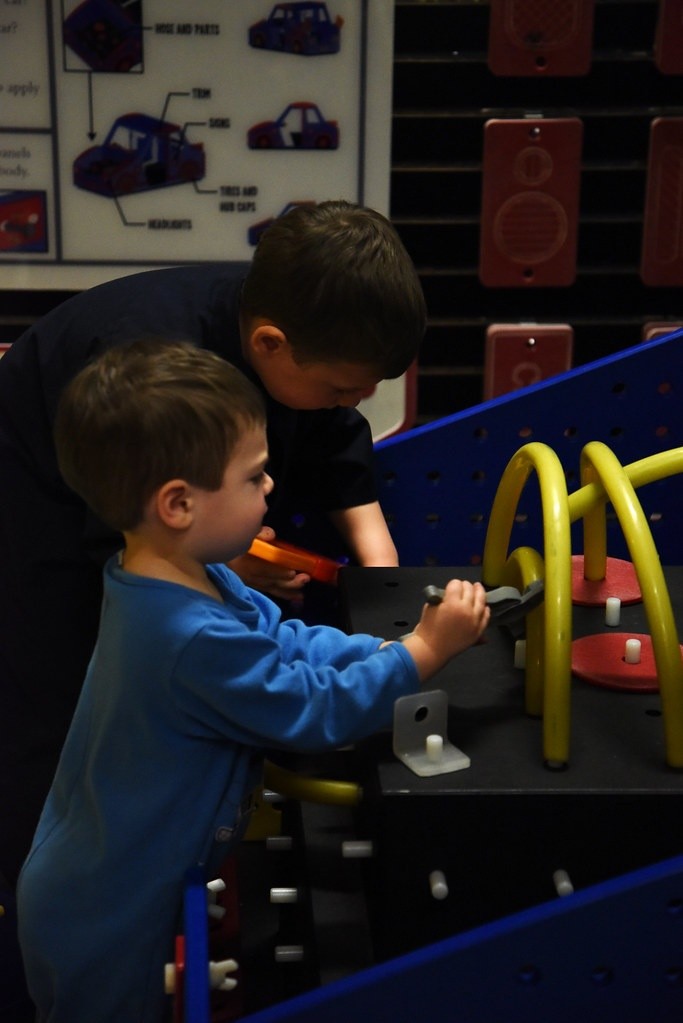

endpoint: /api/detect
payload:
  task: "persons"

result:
[15,344,490,1023]
[0,193,429,1022]
